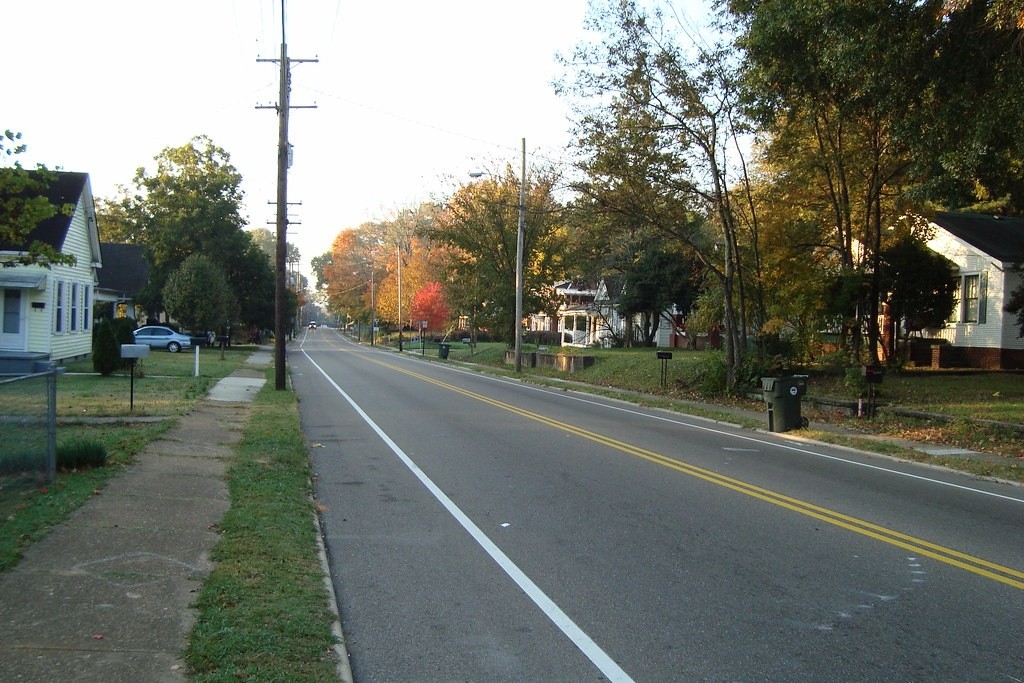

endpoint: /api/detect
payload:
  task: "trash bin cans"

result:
[438,343,451,360]
[760,375,810,432]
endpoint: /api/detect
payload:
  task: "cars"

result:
[132,325,191,353]
[308,321,316,330]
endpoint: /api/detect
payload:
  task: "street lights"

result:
[468,171,526,371]
